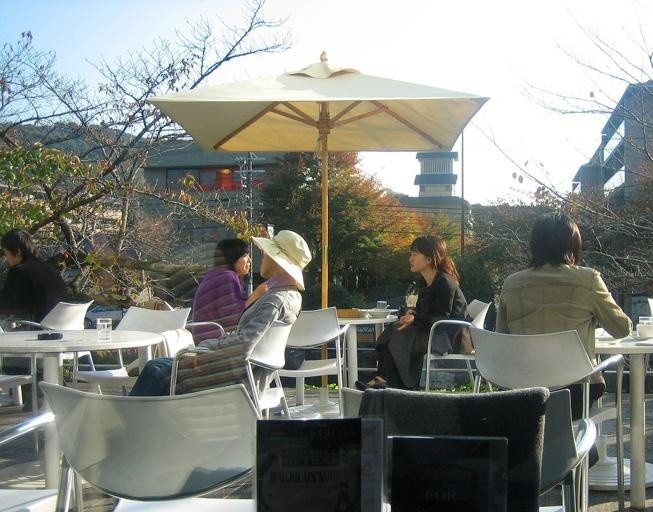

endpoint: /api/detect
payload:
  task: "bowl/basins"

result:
[367,309,391,318]
[636,324,652,338]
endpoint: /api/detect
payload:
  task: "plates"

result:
[596,336,613,341]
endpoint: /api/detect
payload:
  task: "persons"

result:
[128,230,310,395]
[189,236,268,344]
[355,234,469,391]
[492,211,634,421]
[0,228,67,324]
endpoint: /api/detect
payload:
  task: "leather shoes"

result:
[354,375,389,391]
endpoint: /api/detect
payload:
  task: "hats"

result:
[250,229,313,292]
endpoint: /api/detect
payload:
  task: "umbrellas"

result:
[144,50,489,400]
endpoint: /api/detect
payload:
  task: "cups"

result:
[95,317,113,343]
[376,301,387,310]
[636,317,652,325]
